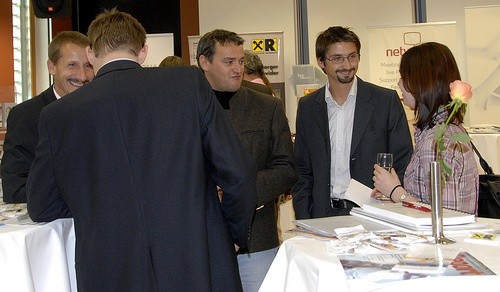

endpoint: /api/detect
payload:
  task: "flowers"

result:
[436,80,473,184]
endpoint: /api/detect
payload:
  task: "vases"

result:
[431,162,454,246]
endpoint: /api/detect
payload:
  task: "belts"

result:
[330,198,357,209]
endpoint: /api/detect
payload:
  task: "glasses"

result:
[325,52,360,64]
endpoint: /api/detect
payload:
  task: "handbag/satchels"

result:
[465,128,500,219]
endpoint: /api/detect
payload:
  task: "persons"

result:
[370,41,480,218]
[292,25,413,220]
[27,6,256,292]
[0,30,95,204]
[158,28,294,292]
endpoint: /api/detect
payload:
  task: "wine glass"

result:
[376,153,392,201]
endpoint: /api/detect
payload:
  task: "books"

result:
[284,200,499,283]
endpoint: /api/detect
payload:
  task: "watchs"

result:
[397,192,407,203]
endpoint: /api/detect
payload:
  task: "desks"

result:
[0,203,77,292]
[259,218,500,292]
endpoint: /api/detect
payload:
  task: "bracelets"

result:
[389,185,409,203]
